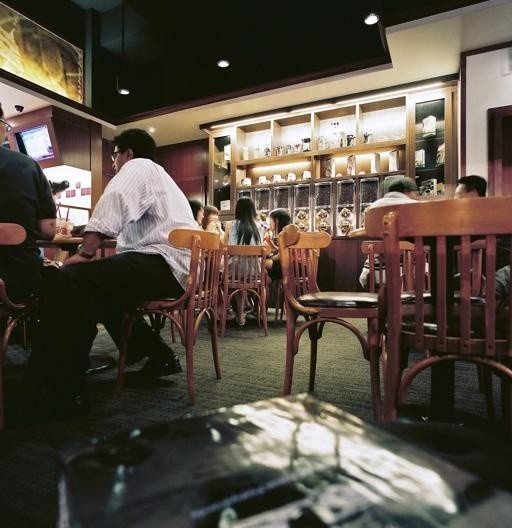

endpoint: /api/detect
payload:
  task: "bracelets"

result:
[76,244,96,259]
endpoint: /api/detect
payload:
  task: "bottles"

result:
[243,130,369,159]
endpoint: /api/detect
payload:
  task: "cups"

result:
[55,218,72,237]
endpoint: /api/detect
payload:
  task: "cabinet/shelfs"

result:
[235,96,409,186]
[406,80,459,199]
[205,125,248,213]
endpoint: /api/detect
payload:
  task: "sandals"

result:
[238,316,246,325]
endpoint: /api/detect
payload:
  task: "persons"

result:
[222,196,263,328]
[451,175,511,307]
[201,205,235,324]
[188,198,203,225]
[33,127,206,418]
[358,174,430,292]
[261,207,298,308]
[0,102,56,304]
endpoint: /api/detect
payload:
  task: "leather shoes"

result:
[123,332,182,385]
[33,389,89,418]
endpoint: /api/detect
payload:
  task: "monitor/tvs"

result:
[7,116,65,168]
